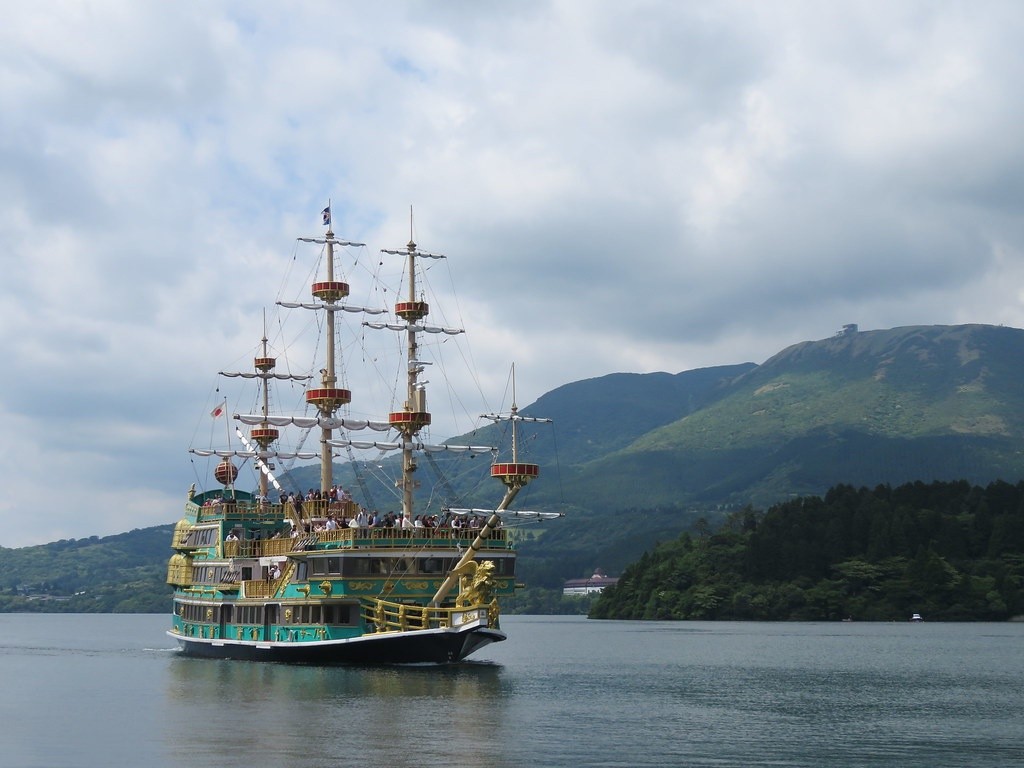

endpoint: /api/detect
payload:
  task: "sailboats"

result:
[166,196,566,665]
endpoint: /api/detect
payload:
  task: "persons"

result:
[402,514,448,548]
[450,515,504,549]
[348,507,403,548]
[225,531,239,558]
[290,525,299,538]
[278,485,347,518]
[202,494,237,513]
[271,565,281,580]
[304,515,351,551]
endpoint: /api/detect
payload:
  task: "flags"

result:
[320,207,331,225]
[210,400,226,421]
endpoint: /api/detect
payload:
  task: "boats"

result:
[910,613,923,622]
